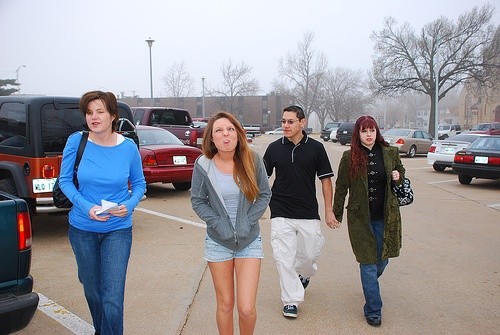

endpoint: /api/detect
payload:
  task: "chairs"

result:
[491,144,500,150]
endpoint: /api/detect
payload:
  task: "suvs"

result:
[0,94,140,225]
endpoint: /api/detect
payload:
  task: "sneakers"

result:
[283,305,297,318]
[367,317,381,325]
[299,274,310,289]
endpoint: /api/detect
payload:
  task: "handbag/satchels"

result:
[53,131,89,208]
[391,177,414,206]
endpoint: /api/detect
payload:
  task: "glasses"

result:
[280,119,299,124]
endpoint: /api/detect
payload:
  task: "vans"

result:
[130,107,199,147]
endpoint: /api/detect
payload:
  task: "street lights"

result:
[145,37,157,106]
[200,76,206,117]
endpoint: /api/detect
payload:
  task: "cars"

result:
[135,124,204,192]
[452,135,500,185]
[192,117,211,138]
[438,125,456,140]
[426,134,492,171]
[466,122,500,134]
[320,121,355,145]
[382,129,435,158]
[265,127,283,136]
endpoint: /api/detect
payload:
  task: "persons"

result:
[263,105,338,318]
[191,110,272,335]
[59,91,146,335]
[332,116,406,326]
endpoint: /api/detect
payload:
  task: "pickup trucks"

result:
[0,191,41,335]
